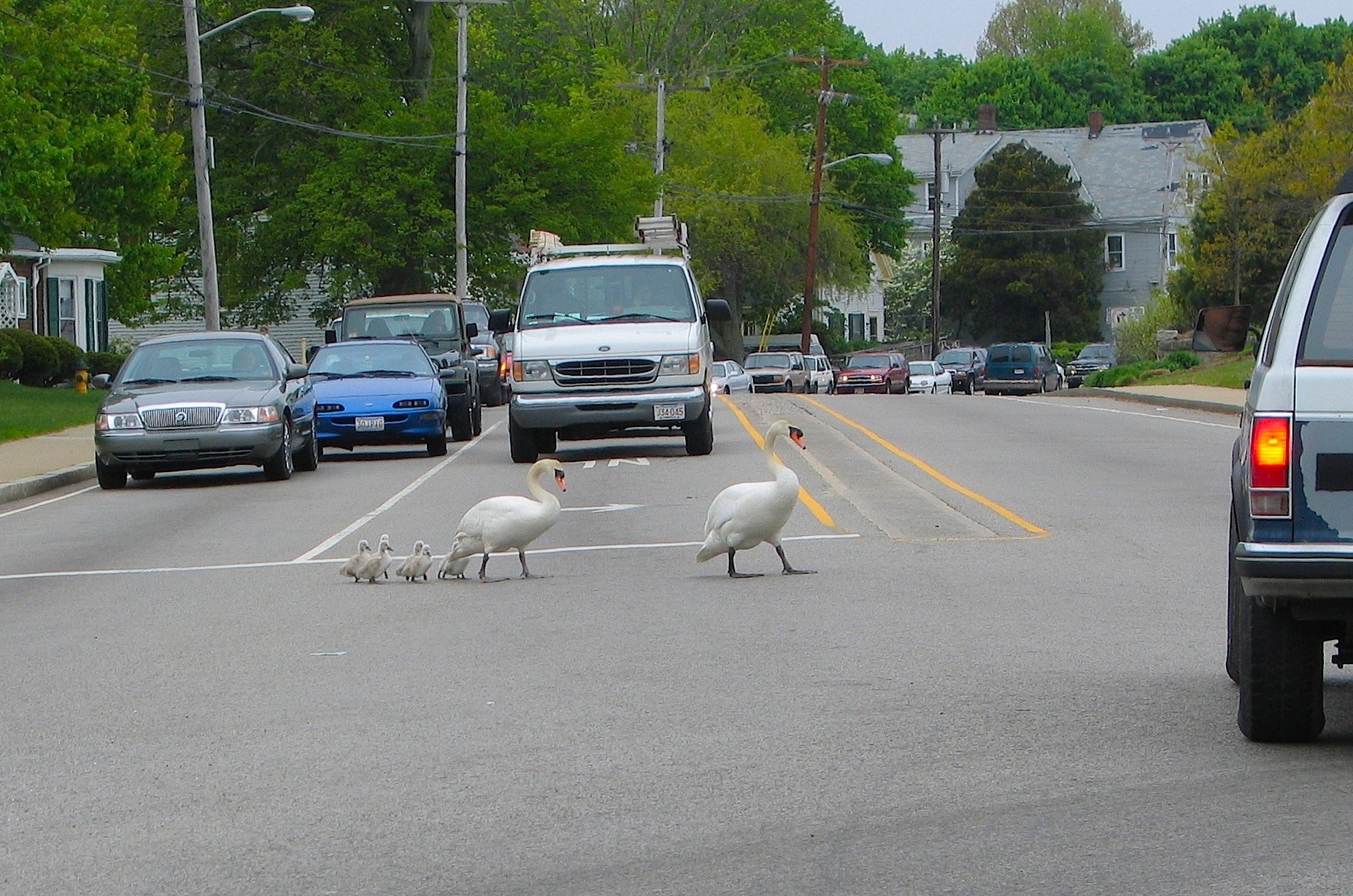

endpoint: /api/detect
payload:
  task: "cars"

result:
[710,359,756,398]
[907,360,953,396]
[296,339,456,461]
[91,329,320,489]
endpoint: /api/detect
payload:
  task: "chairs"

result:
[367,319,391,336]
[155,357,180,376]
[535,292,578,315]
[421,321,446,332]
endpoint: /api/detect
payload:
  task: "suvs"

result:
[743,351,811,394]
[322,291,484,442]
[457,300,515,408]
[1066,343,1114,391]
[1225,189,1353,746]
[834,349,910,395]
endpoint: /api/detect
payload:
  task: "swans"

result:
[395,541,433,582]
[448,458,566,583]
[339,540,371,584]
[438,541,470,579]
[695,420,819,579]
[357,534,394,583]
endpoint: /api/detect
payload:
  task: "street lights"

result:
[184,0,315,330]
[800,153,893,351]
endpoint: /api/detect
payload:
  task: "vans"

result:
[804,354,835,396]
[744,333,824,356]
[932,347,988,397]
[986,342,1063,396]
[488,252,732,465]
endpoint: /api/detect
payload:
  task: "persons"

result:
[466,311,486,331]
[1193,306,1250,352]
[233,347,259,373]
[260,325,270,338]
[399,344,418,369]
[629,280,662,308]
[424,310,448,334]
